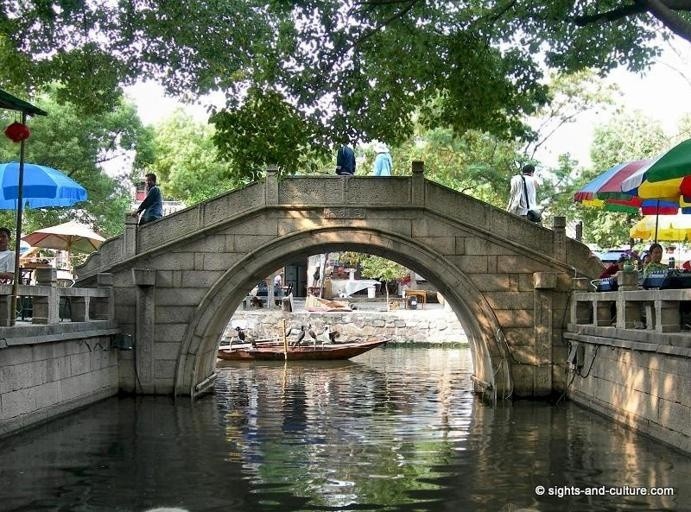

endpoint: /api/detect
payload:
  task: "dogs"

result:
[336,289,353,298]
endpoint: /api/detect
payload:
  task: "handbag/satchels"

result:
[526,209,542,222]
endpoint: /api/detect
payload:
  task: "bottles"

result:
[29,258,34,268]
[648,269,668,287]
[36,258,41,266]
[668,257,677,269]
[601,277,615,290]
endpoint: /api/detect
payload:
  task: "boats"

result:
[217,326,393,362]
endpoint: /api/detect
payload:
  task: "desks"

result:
[404,288,428,311]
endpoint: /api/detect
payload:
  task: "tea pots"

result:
[42,258,51,264]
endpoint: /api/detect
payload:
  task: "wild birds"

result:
[308,323,317,344]
[322,325,340,343]
[291,325,305,350]
[234,326,246,343]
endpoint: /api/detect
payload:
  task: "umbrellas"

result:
[620,138,691,200]
[574,159,691,244]
[630,208,691,243]
[19,221,106,270]
[0,161,88,211]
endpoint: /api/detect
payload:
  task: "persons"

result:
[507,165,542,224]
[274,274,284,296]
[599,244,691,278]
[131,174,163,227]
[235,327,245,343]
[400,274,411,305]
[371,143,392,176]
[336,143,356,175]
[0,228,16,284]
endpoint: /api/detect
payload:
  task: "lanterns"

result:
[4,121,30,143]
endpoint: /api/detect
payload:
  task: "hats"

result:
[375,145,389,153]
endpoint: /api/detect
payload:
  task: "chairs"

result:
[2,264,77,322]
[383,281,404,310]
